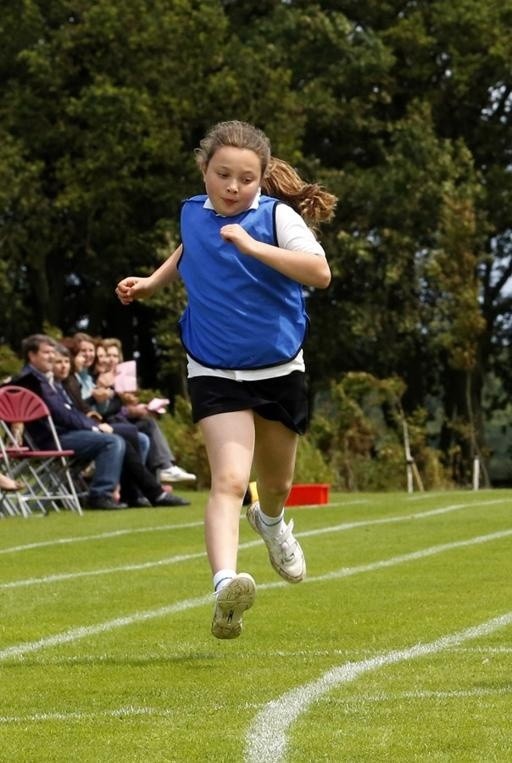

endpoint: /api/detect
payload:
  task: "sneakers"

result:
[210,572,257,640]
[157,464,197,483]
[245,500,306,584]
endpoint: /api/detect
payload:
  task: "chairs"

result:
[1,385,93,516]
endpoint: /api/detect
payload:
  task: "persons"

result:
[112,118,340,645]
[1,323,199,510]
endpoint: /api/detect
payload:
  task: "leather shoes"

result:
[150,493,191,506]
[86,495,128,509]
[1,479,25,492]
[119,496,151,506]
